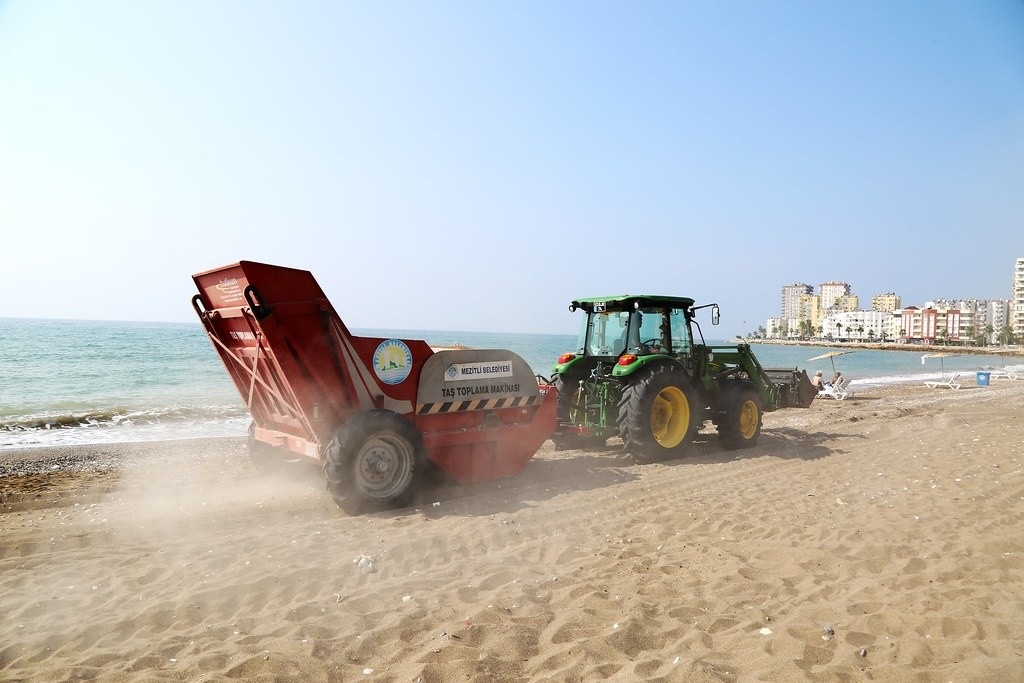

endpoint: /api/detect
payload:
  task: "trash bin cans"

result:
[976,372,991,385]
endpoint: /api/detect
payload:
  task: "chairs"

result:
[924,371,961,390]
[822,378,852,399]
[990,368,1018,383]
[814,376,846,401]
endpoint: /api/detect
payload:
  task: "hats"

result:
[836,372,840,376]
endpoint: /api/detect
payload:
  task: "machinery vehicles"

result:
[551,296,820,463]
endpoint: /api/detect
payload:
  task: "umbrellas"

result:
[923,353,962,380]
[808,351,856,376]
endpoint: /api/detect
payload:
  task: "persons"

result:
[812,371,843,397]
[621,312,644,348]
